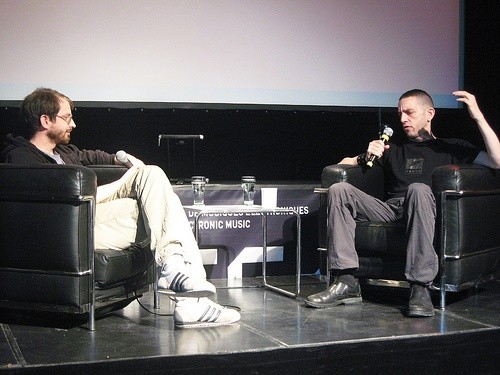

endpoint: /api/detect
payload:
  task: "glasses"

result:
[43,112,73,124]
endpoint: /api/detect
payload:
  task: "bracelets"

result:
[356,153,367,166]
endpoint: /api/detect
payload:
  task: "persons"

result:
[305,87,500,315]
[0,86,241,328]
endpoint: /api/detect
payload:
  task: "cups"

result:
[191,176,206,205]
[241,176,256,205]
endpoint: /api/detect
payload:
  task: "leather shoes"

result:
[406,283,435,316]
[305,281,362,308]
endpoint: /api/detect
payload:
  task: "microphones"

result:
[115,150,133,169]
[367,127,393,168]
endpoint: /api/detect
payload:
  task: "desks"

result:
[184,205,301,298]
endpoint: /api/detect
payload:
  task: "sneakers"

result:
[174,296,242,328]
[157,262,216,296]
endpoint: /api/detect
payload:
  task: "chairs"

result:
[314,164,500,309]
[0,163,160,331]
